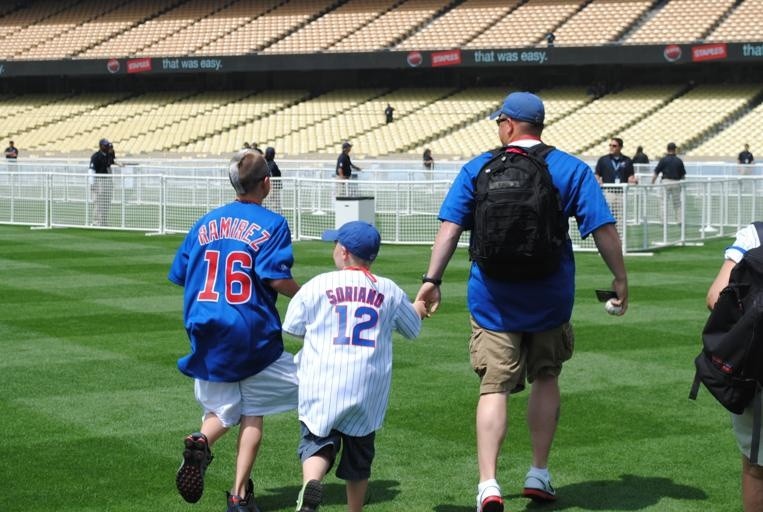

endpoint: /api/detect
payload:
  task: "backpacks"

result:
[470,144,562,283]
[694,221,762,415]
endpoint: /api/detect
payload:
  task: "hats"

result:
[668,143,677,150]
[266,148,275,154]
[99,138,113,149]
[342,143,354,148]
[490,92,544,123]
[322,221,380,260]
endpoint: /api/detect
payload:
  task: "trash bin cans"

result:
[334,197,374,231]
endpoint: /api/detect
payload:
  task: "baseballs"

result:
[606,298,623,315]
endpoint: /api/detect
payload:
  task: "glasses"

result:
[609,143,616,148]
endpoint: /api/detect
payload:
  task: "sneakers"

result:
[176,433,212,503]
[295,480,324,511]
[477,479,503,512]
[523,472,555,501]
[226,481,260,512]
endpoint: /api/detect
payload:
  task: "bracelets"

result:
[421,273,442,285]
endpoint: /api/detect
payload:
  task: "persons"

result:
[167,148,301,512]
[334,141,360,180]
[422,148,433,171]
[631,146,649,163]
[597,135,638,199]
[283,222,427,512]
[415,90,629,512]
[384,103,394,125]
[738,144,754,175]
[243,141,283,190]
[650,141,685,226]
[90,138,122,227]
[4,140,17,158]
[707,223,763,512]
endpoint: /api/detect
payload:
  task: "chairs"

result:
[0,0,762,161]
